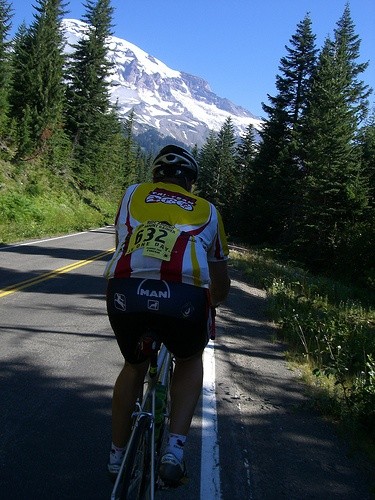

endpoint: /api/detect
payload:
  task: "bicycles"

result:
[111,329,176,500]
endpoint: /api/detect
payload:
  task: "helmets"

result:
[151,144,199,184]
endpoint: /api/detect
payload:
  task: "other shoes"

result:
[158,452,186,488]
[107,463,138,481]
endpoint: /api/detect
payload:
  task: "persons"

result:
[104,145,232,492]
